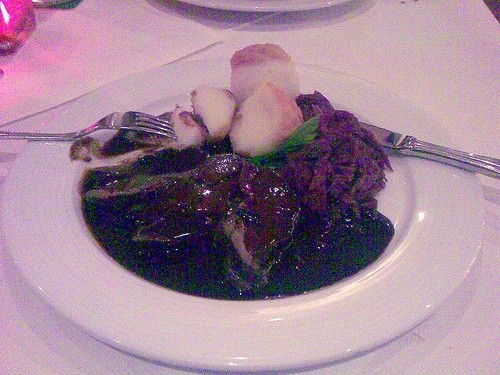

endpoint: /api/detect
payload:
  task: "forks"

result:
[1,111,176,142]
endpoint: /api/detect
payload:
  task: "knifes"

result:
[359,120,500,175]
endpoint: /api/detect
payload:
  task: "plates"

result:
[0,60,485,374]
[179,0,350,13]
